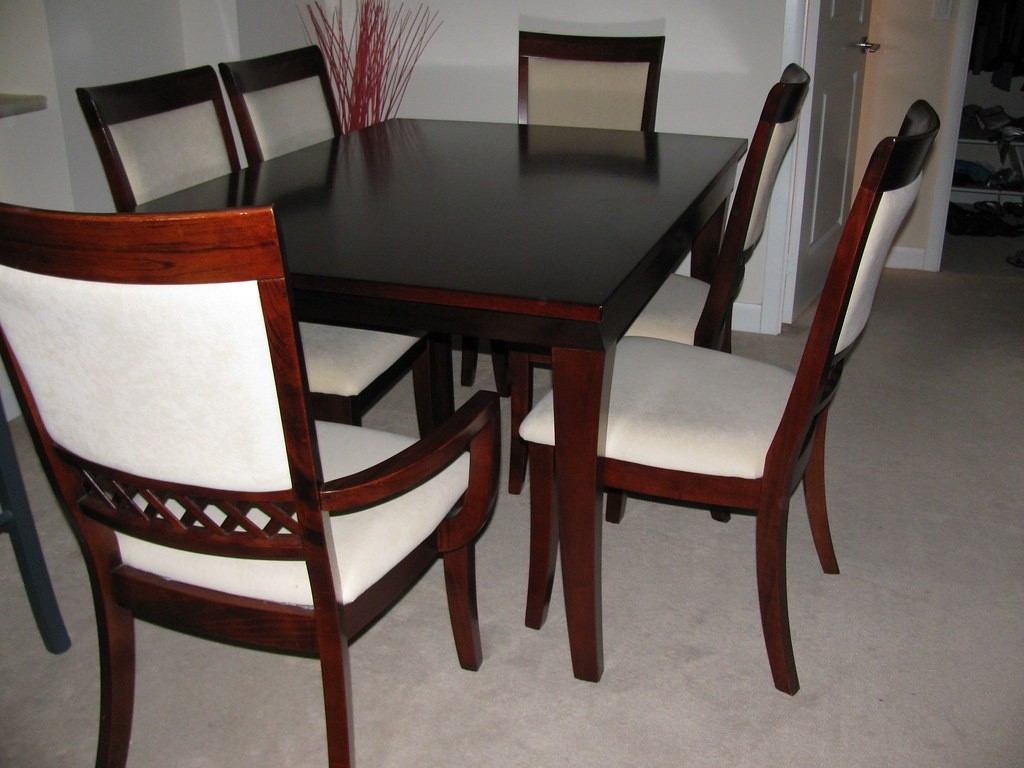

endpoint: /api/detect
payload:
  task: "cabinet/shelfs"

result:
[948,137,1024,213]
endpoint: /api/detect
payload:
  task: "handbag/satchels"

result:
[975,105,1010,131]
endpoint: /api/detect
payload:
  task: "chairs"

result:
[0,202,502,768]
[492,31,668,398]
[507,64,811,527]
[519,98,941,694]
[73,65,434,440]
[217,45,480,415]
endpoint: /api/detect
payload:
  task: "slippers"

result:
[974,200,1004,216]
[1003,202,1024,219]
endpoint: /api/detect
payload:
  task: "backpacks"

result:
[946,210,1024,239]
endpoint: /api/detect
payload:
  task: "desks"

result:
[113,117,750,685]
[0,95,71,655]
